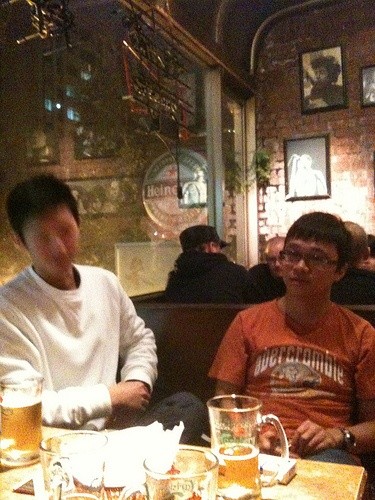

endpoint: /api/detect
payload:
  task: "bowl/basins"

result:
[72,463,177,500]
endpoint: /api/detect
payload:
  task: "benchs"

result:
[135,302,375,397]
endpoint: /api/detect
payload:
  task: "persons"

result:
[164,224,266,304]
[304,53,342,107]
[1,172,208,445]
[208,214,375,466]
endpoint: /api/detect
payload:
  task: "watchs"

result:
[338,424,357,451]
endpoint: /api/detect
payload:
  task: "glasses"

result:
[280,248,343,266]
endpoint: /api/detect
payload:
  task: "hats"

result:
[179,225,227,250]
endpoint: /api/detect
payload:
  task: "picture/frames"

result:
[283,134,331,201]
[299,44,347,115]
[359,64,375,109]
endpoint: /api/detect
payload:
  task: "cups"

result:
[38,429,110,500]
[206,393,290,500]
[0,374,45,468]
[116,444,220,500]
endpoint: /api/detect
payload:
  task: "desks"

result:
[0,425,367,500]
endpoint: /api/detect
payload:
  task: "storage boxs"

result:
[259,454,297,485]
